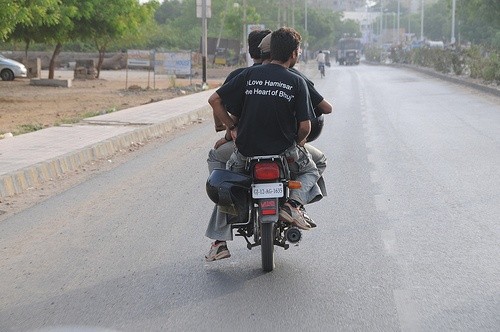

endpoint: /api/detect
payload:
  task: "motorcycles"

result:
[205,111,325,273]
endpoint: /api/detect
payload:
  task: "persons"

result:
[213,30,332,149]
[207,32,327,228]
[205,27,323,262]
[316,51,325,77]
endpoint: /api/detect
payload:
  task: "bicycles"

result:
[316,59,326,78]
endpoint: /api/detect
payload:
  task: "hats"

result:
[258,33,271,53]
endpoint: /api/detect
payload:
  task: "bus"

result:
[336,32,363,66]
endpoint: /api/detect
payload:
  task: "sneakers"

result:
[280,200,311,232]
[301,211,318,228]
[200,239,231,262]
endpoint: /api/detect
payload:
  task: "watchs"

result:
[225,134,233,142]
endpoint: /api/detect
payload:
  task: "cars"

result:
[0,54,28,81]
